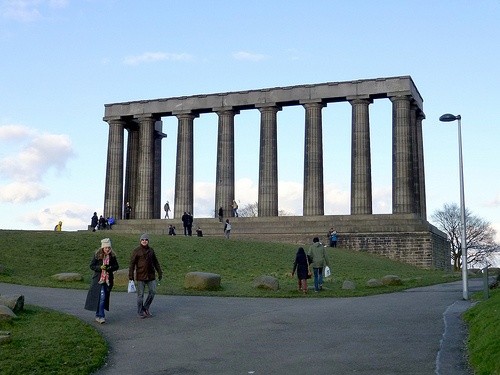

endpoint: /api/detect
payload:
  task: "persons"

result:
[182,212,192,236]
[91,212,115,232]
[224,219,231,239]
[164,201,170,219]
[55,221,62,230]
[291,247,313,294]
[85,238,120,323]
[232,200,238,217]
[327,229,337,247]
[129,233,162,318]
[196,227,203,237]
[125,201,133,219]
[307,237,330,292]
[218,207,224,223]
[169,225,175,236]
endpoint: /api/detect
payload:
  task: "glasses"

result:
[141,239,148,240]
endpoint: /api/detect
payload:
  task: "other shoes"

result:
[136,311,144,319]
[314,290,318,293]
[319,285,322,289]
[143,306,152,317]
[96,317,105,324]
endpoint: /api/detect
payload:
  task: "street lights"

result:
[438,113,469,301]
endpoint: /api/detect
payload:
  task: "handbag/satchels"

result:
[128,280,136,293]
[325,266,330,277]
[308,271,312,278]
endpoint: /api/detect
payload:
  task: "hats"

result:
[140,233,149,240]
[101,238,112,247]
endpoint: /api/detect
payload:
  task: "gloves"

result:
[100,264,110,272]
[158,273,162,281]
[129,276,134,281]
